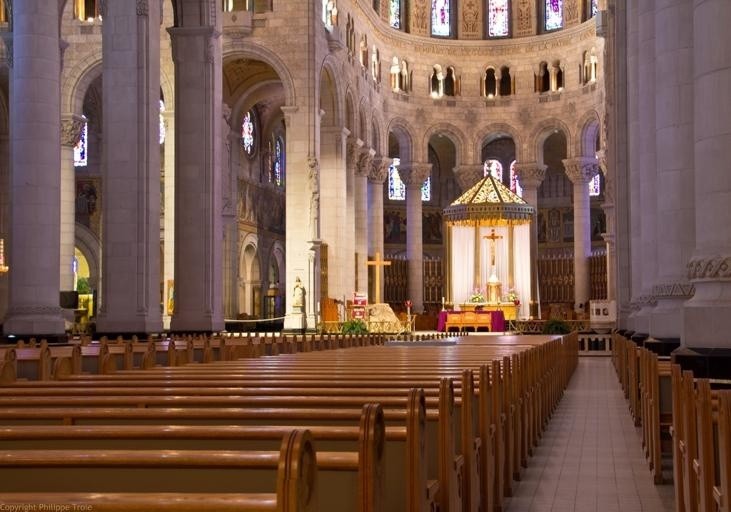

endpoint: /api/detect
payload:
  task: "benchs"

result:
[603,322,731,512]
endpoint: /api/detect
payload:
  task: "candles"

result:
[441,296,445,304]
